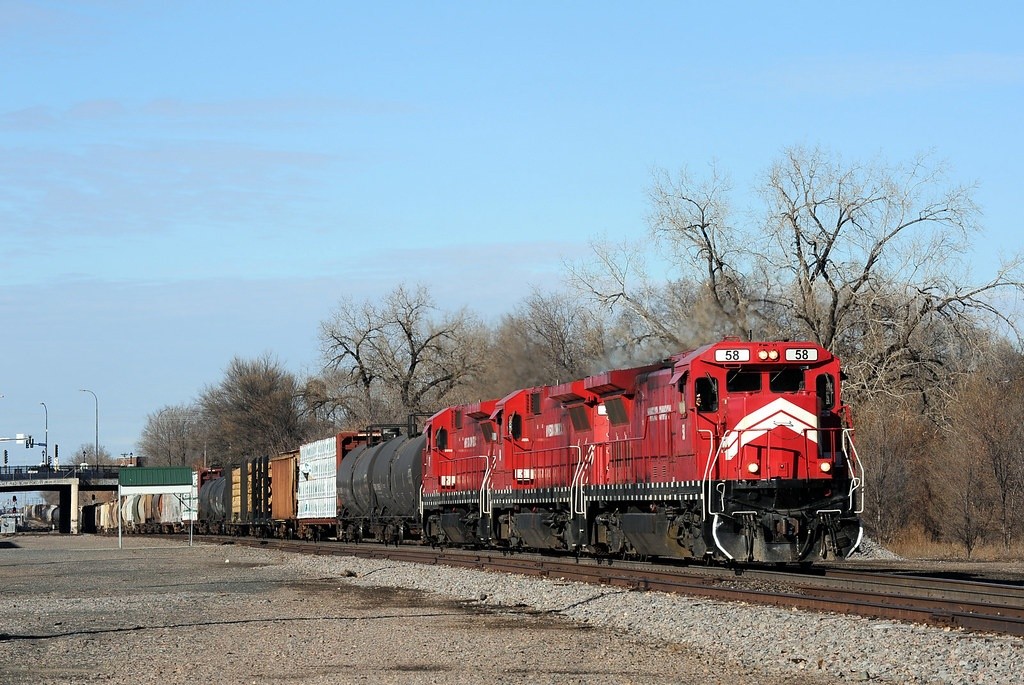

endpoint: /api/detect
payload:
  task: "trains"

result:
[18,341,869,576]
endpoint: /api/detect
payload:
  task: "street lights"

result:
[38,402,55,466]
[78,388,99,471]
